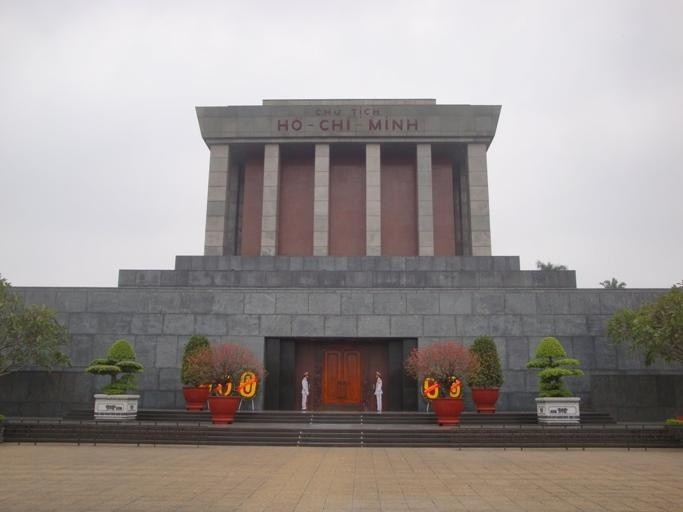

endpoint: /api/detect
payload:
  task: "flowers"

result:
[190,344,269,395]
[400,339,484,397]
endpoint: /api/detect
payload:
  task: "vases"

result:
[430,395,464,428]
[204,393,240,425]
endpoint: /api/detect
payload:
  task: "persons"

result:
[300,372,310,411]
[374,370,383,415]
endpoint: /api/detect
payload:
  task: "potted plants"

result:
[523,335,586,425]
[84,336,144,422]
[466,333,504,414]
[179,335,213,412]
